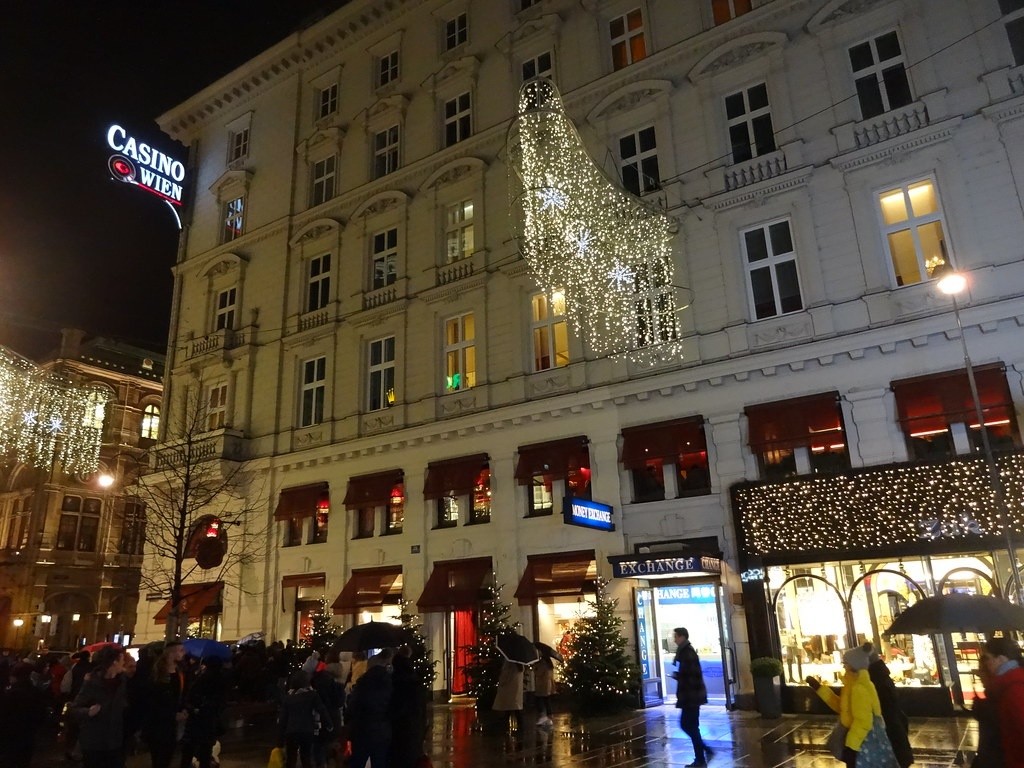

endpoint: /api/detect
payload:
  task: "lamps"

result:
[925,256,945,276]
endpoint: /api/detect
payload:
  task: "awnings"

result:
[330,565,402,608]
[153,581,223,619]
[415,557,492,606]
[514,550,594,598]
[271,422,708,521]
[745,398,846,454]
[896,368,1012,431]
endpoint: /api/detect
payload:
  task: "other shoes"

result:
[512,726,529,736]
[705,747,716,762]
[536,716,553,726]
[65,750,84,762]
[685,758,707,768]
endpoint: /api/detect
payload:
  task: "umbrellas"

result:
[496,634,539,665]
[884,592,1024,635]
[331,620,411,652]
[535,641,564,663]
[182,638,230,660]
[83,642,119,652]
[236,632,267,644]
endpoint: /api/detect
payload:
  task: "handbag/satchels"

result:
[856,713,900,768]
[334,735,352,759]
[59,663,78,698]
[267,747,286,768]
[410,752,433,768]
[55,700,74,723]
[826,721,848,761]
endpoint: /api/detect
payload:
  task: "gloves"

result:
[839,746,858,764]
[806,676,821,690]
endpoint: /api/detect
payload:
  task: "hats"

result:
[392,646,412,667]
[843,641,873,670]
[352,641,369,659]
[868,643,880,664]
[301,651,320,673]
[367,647,392,668]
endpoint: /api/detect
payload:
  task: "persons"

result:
[787,633,803,683]
[492,659,524,736]
[670,628,714,768]
[536,654,555,725]
[806,641,915,768]
[964,638,1024,768]
[0,635,427,768]
